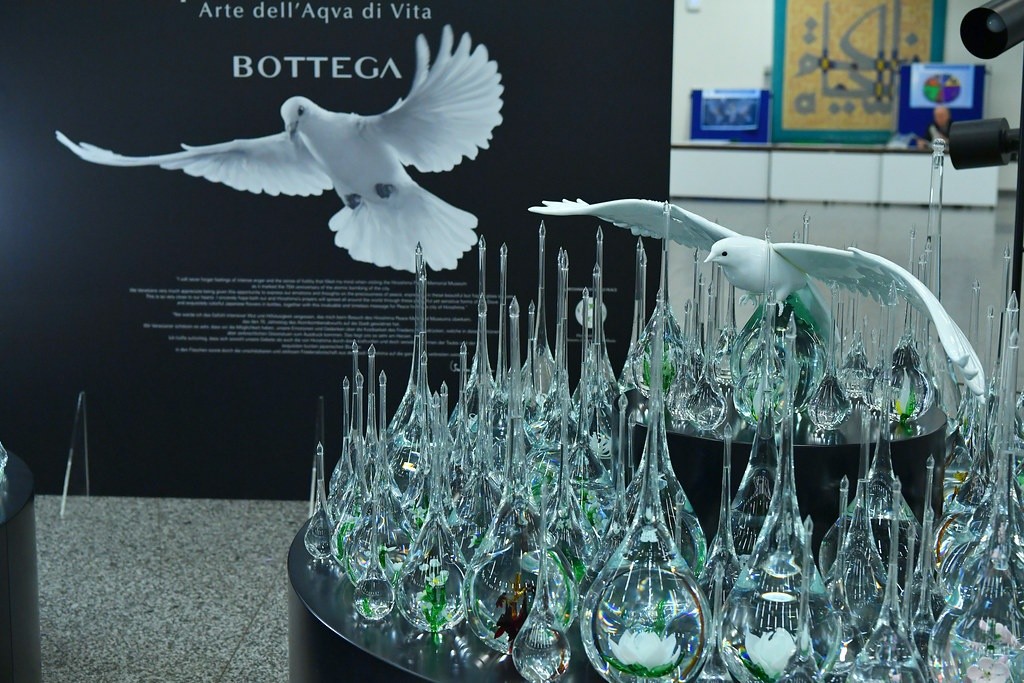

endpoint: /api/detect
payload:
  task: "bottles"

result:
[304,132,1024,683]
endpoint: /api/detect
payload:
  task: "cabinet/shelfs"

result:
[665,140,1016,378]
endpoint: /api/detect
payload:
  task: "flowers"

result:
[288,341,1024,683]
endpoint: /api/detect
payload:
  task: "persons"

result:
[705,101,758,125]
[918,106,955,151]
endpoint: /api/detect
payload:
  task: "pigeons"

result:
[56,25,503,274]
[528,198,986,408]
